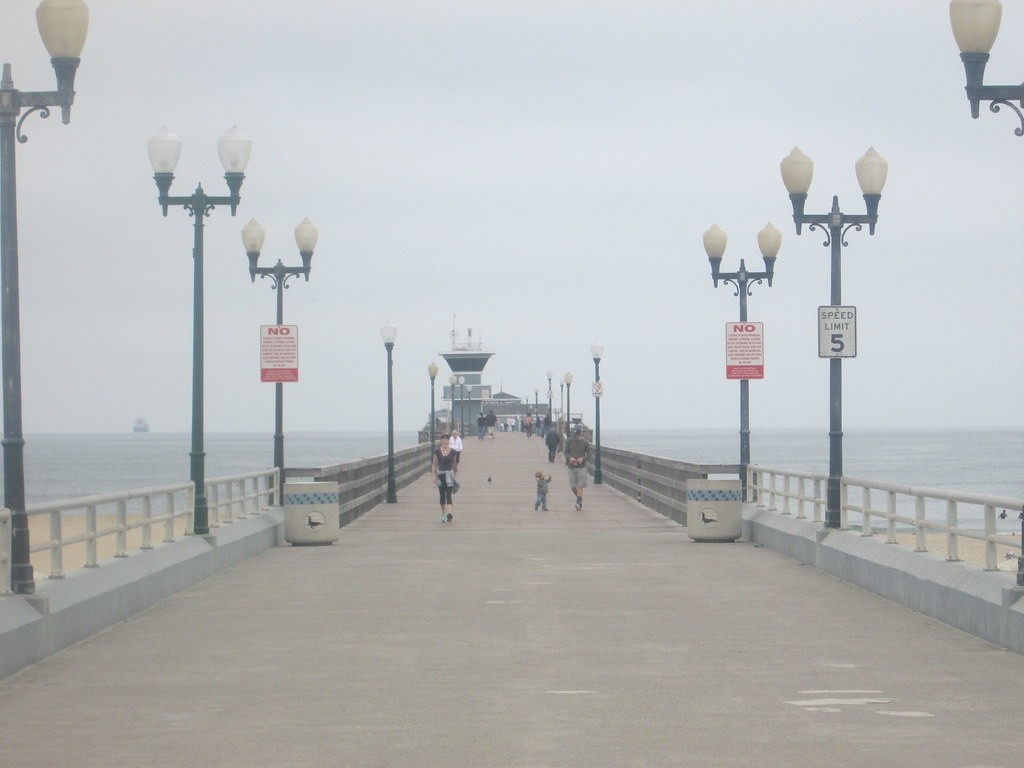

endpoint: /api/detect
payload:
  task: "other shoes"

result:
[441,514,447,521]
[446,512,452,522]
[535,504,538,510]
[542,507,549,511]
[576,497,583,510]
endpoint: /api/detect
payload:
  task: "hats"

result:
[573,427,584,437]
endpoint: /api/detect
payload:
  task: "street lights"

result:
[525,395,529,419]
[563,371,573,433]
[558,378,564,421]
[147,116,252,534]
[239,215,318,507]
[466,385,473,434]
[533,388,539,419]
[449,373,458,434]
[458,376,466,437]
[545,367,553,421]
[380,317,399,504]
[779,139,889,527]
[701,219,783,504]
[1,0,92,597]
[590,337,603,484]
[429,361,439,465]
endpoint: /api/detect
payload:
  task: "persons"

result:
[524,413,533,439]
[486,410,497,439]
[478,412,486,440]
[564,429,592,510]
[432,433,458,522]
[545,427,559,463]
[535,414,550,438]
[534,471,552,511]
[500,421,508,431]
[511,418,516,432]
[448,429,463,471]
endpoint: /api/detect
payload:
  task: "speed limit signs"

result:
[818,305,857,359]
